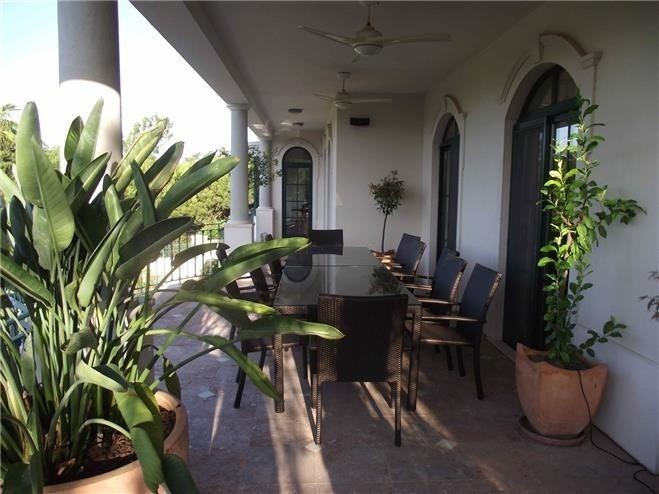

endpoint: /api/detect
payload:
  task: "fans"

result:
[295,1,452,63]
[313,72,392,111]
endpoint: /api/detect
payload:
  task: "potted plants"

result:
[514,83,648,439]
[367,169,406,256]
[0,96,347,494]
